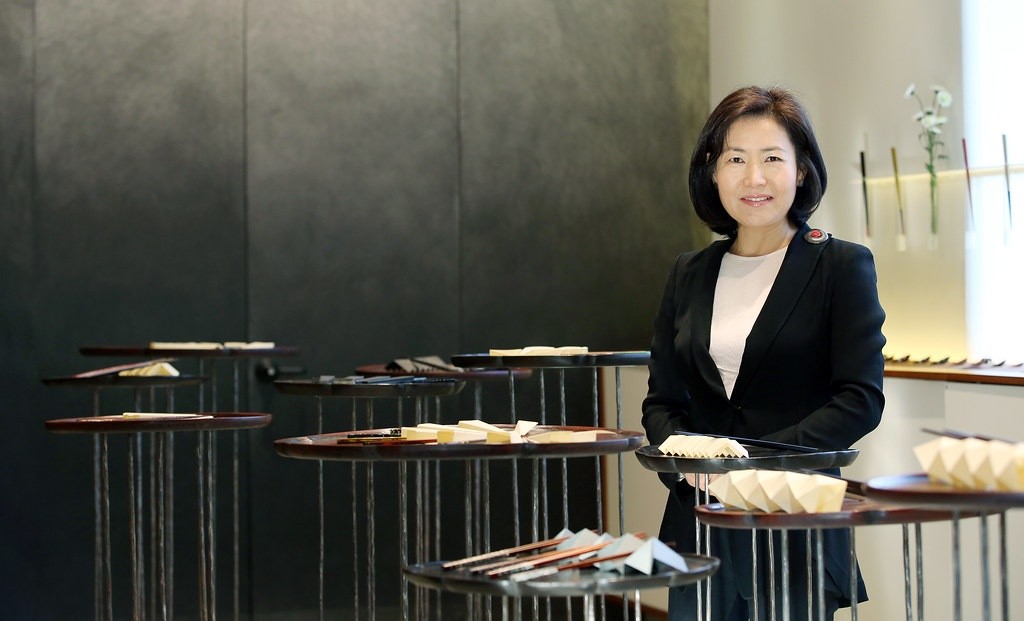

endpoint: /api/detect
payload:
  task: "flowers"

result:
[905,80,954,233]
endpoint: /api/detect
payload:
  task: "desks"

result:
[40,337,1023,621]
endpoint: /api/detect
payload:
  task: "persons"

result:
[642,85,886,621]
